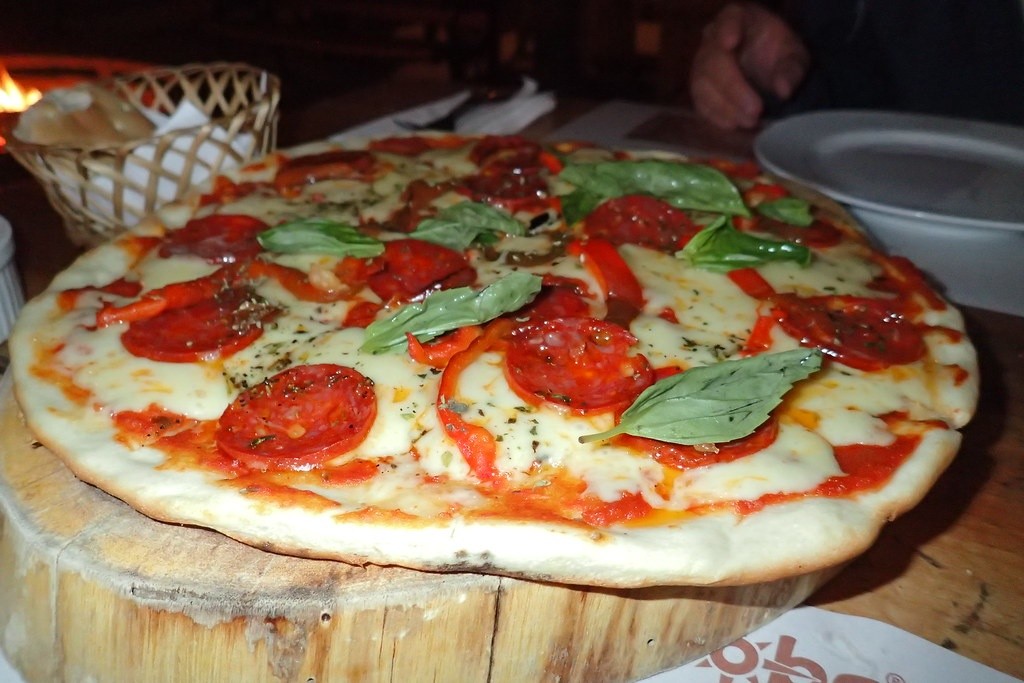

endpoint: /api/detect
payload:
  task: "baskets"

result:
[2,61,282,253]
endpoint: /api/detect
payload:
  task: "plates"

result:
[756,110,1023,241]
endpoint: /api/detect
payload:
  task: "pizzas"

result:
[8,131,981,589]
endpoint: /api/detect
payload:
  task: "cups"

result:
[0,218,28,341]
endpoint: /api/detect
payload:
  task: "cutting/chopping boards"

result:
[1,359,859,683]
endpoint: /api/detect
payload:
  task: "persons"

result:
[688,1,1023,133]
